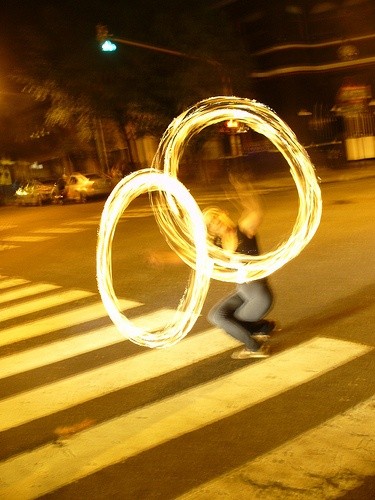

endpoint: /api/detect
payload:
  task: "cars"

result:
[12,182,59,207]
[49,172,114,204]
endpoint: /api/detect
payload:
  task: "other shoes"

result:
[253,319,282,342]
[230,344,272,359]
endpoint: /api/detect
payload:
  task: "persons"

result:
[141,167,279,361]
